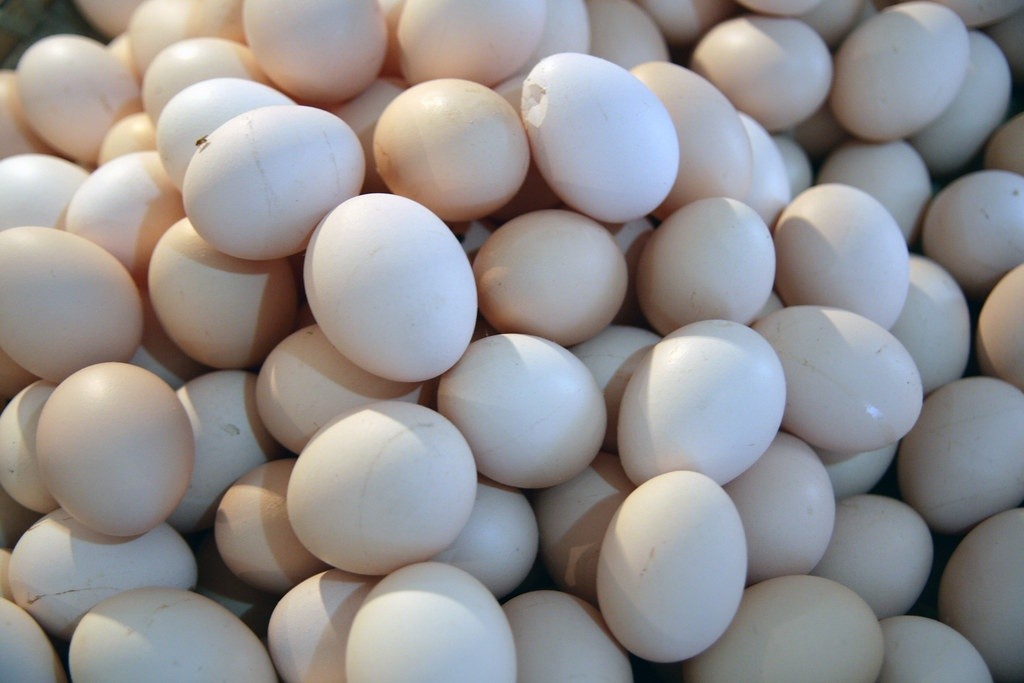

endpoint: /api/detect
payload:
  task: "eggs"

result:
[0,0,1024,683]
